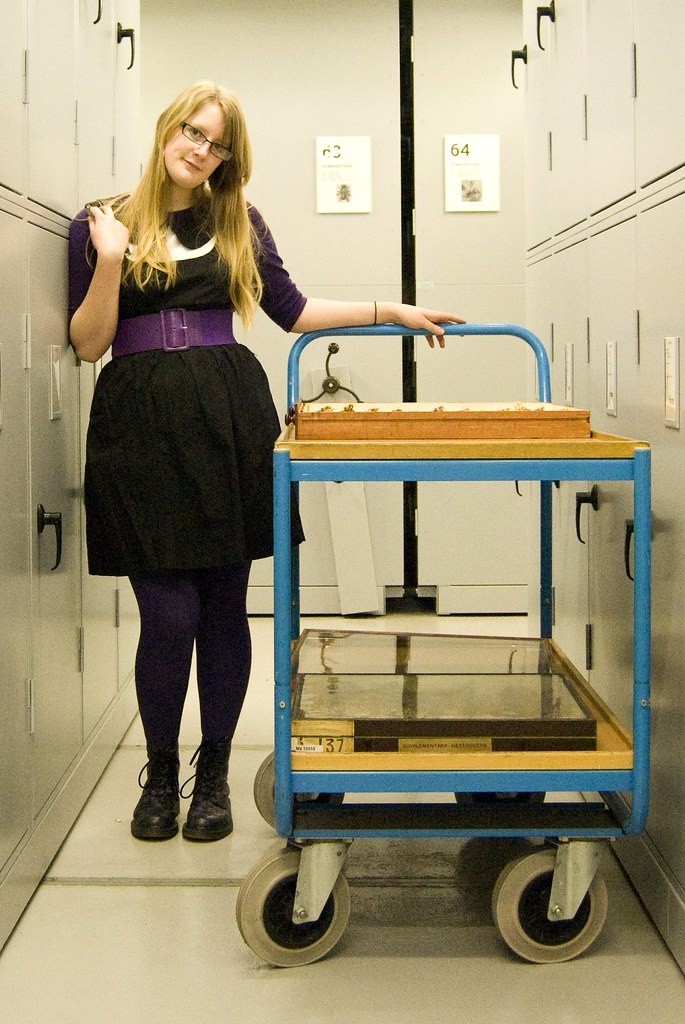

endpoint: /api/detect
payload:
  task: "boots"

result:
[130,733,180,838]
[180,735,233,839]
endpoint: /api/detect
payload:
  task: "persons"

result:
[68,80,467,841]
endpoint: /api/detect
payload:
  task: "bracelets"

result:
[375,301,377,325]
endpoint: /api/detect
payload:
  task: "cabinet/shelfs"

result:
[510,0,685,979]
[0,0,140,961]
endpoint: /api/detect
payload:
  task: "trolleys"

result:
[233,327,650,968]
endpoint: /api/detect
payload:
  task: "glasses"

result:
[180,121,235,162]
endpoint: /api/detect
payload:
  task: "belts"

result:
[112,306,237,357]
